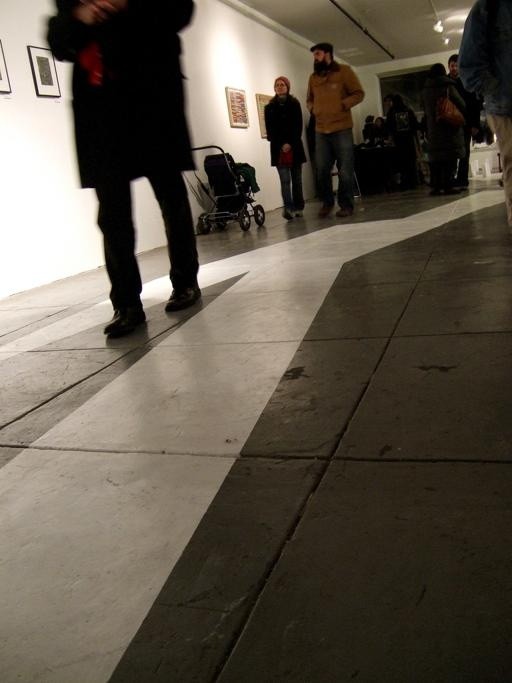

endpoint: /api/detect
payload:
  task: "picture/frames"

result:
[27,44,62,98]
[225,85,250,128]
[255,92,277,138]
[0,38,14,94]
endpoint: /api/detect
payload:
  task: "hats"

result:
[310,43,333,52]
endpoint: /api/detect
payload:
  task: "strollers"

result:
[184,145,266,232]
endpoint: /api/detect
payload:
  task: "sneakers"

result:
[336,208,352,216]
[319,207,329,216]
[283,208,303,220]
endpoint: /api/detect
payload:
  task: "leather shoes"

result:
[103,305,146,332]
[166,287,203,311]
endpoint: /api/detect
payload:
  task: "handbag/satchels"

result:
[440,98,464,122]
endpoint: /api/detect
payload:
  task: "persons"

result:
[45,1,202,340]
[305,42,366,218]
[363,0,512,232]
[265,76,306,220]
